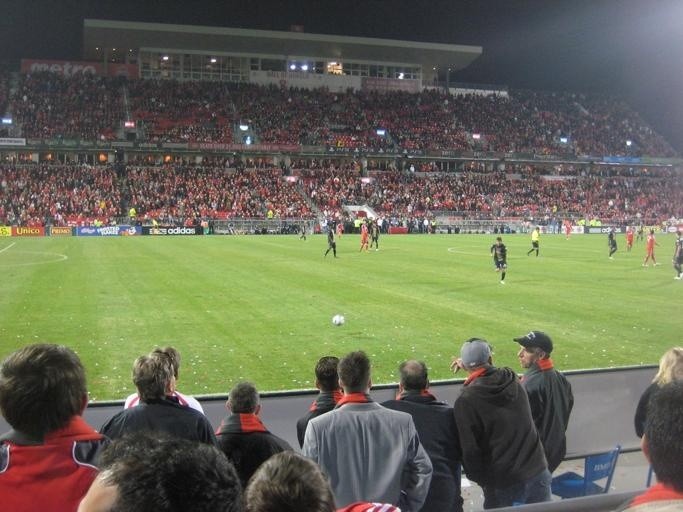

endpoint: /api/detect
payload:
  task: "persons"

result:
[615,380,683,512]
[0,343,112,511]
[635,348,683,438]
[608,225,617,260]
[0,64,683,240]
[526,227,541,257]
[512,331,574,473]
[244,450,402,512]
[377,359,465,512]
[490,237,507,284]
[625,225,633,253]
[450,338,552,510]
[641,230,661,267]
[303,350,434,512]
[671,231,683,280]
[78,347,345,511]
[359,219,380,253]
[323,224,337,258]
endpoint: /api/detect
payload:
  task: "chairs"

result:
[551,443,621,500]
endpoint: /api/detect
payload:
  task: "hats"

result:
[461,338,495,369]
[513,330,553,353]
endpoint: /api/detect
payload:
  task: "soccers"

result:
[333,314,344,326]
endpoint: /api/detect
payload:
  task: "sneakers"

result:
[673,272,683,280]
